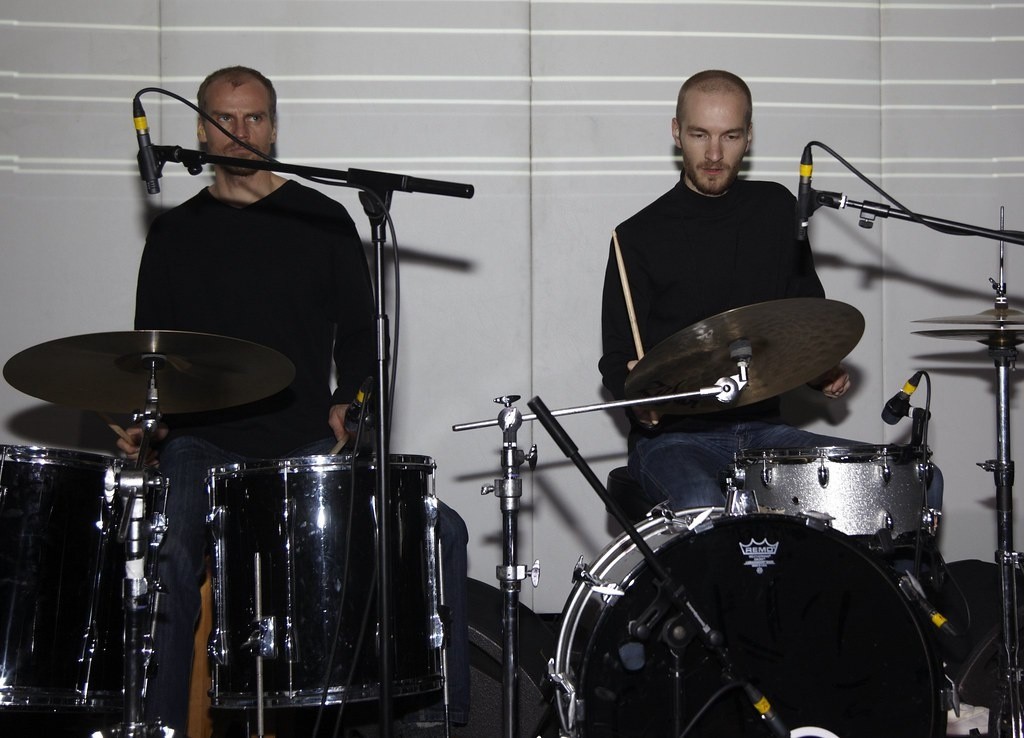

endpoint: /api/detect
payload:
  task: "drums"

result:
[733,447,932,549]
[548,506,948,738]
[0,442,172,705]
[202,452,445,710]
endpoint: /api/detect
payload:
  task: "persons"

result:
[597,67,946,577]
[117,61,469,738]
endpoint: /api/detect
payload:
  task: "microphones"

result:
[794,146,812,242]
[132,98,160,195]
[881,370,922,425]
[343,376,375,433]
[896,576,957,640]
[743,683,791,738]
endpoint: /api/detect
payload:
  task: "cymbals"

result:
[908,308,1024,346]
[2,329,298,416]
[625,298,866,423]
